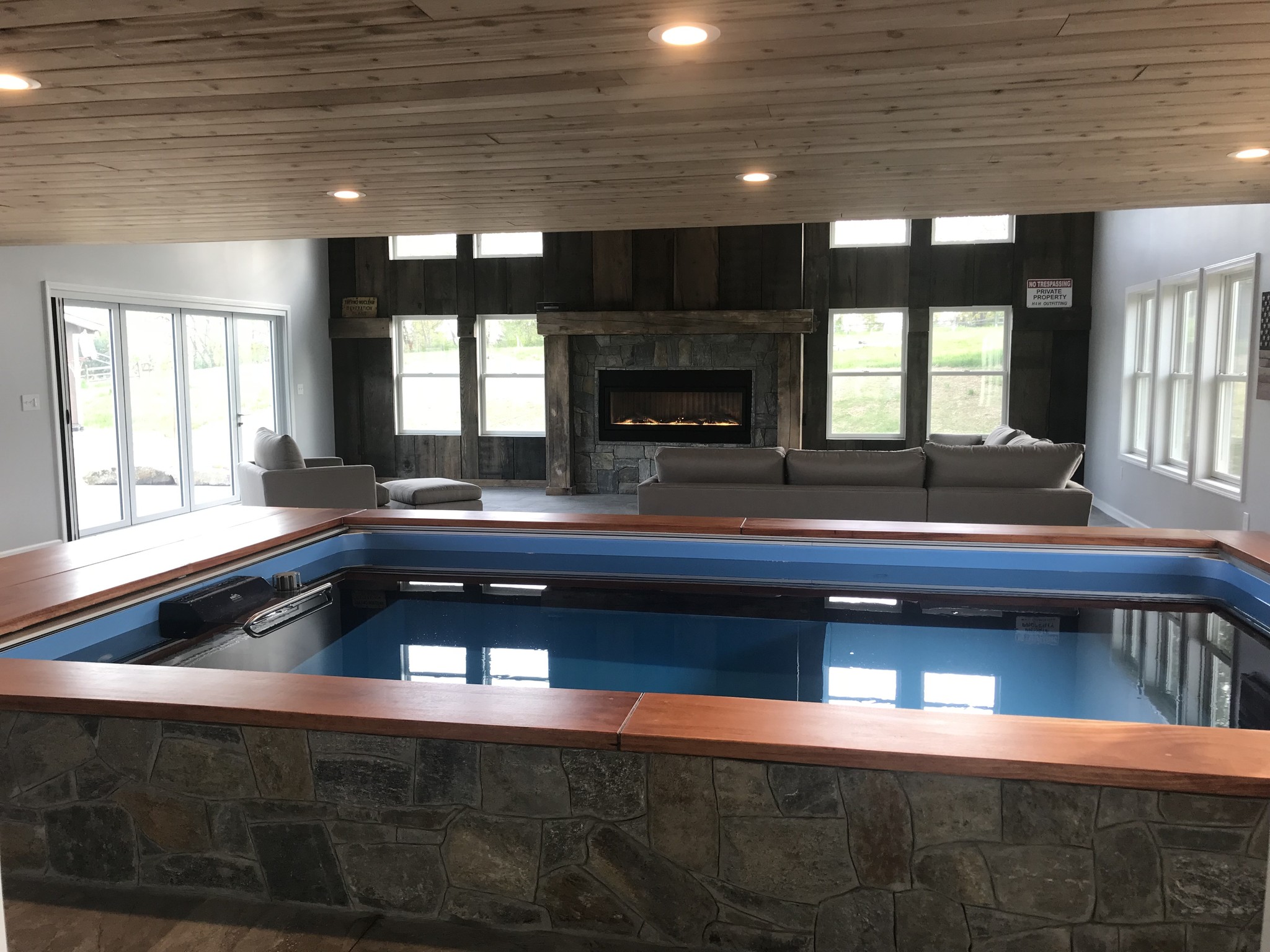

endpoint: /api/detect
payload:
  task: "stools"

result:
[381,477,484,511]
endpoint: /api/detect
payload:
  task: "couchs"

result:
[236,427,393,510]
[638,425,1093,527]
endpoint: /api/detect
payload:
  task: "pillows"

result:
[981,424,1017,446]
[653,446,786,485]
[923,441,1086,490]
[930,433,982,448]
[1035,439,1086,488]
[254,427,308,469]
[786,446,927,489]
[1005,435,1044,446]
